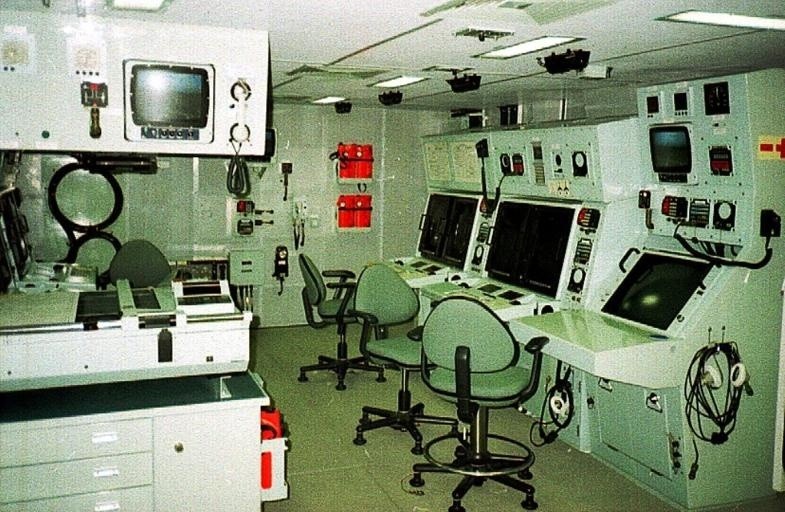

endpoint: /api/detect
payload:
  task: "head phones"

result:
[549,378,574,417]
[697,343,751,391]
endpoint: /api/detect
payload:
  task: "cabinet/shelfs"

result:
[1,371,289,511]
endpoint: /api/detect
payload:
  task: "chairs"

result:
[102,240,171,288]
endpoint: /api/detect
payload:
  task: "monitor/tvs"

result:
[415,191,484,272]
[599,250,716,333]
[483,198,582,300]
[647,122,700,187]
[0,187,34,282]
[123,58,216,145]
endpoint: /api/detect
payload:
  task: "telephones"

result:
[301,200,308,222]
[292,196,301,226]
